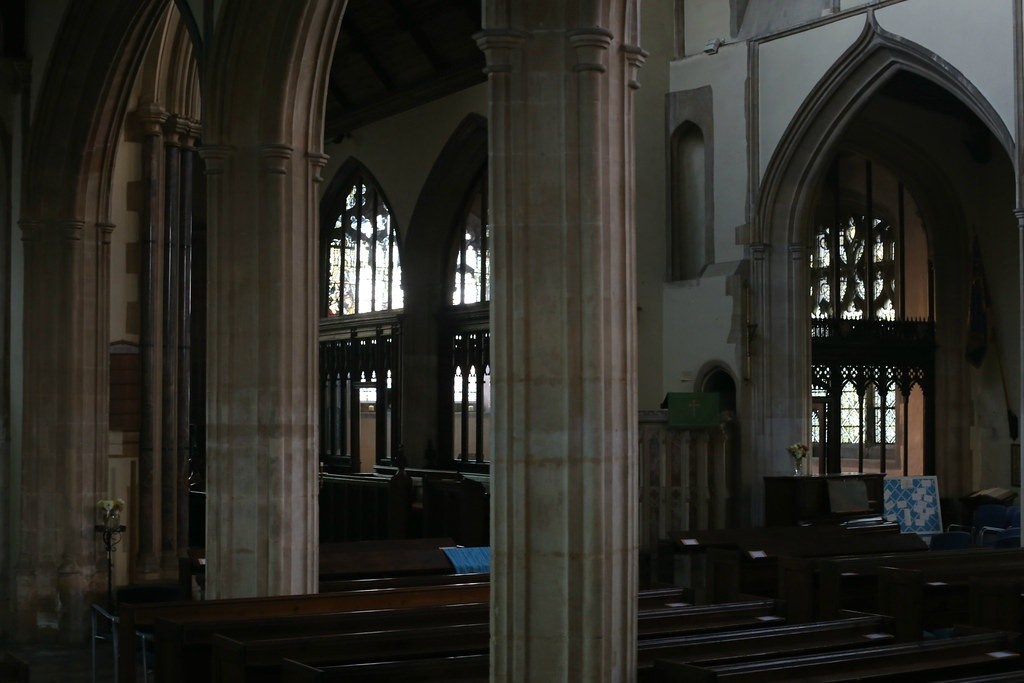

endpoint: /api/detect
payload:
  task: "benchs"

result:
[121,466,1024,683]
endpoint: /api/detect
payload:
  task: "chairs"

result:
[981,505,1021,548]
[972,503,1007,542]
[930,530,973,551]
[91,585,176,683]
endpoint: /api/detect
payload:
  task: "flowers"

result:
[786,442,809,469]
[97,498,125,517]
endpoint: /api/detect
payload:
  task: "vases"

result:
[103,510,120,528]
[792,457,802,476]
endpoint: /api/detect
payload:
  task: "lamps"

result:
[704,38,725,55]
